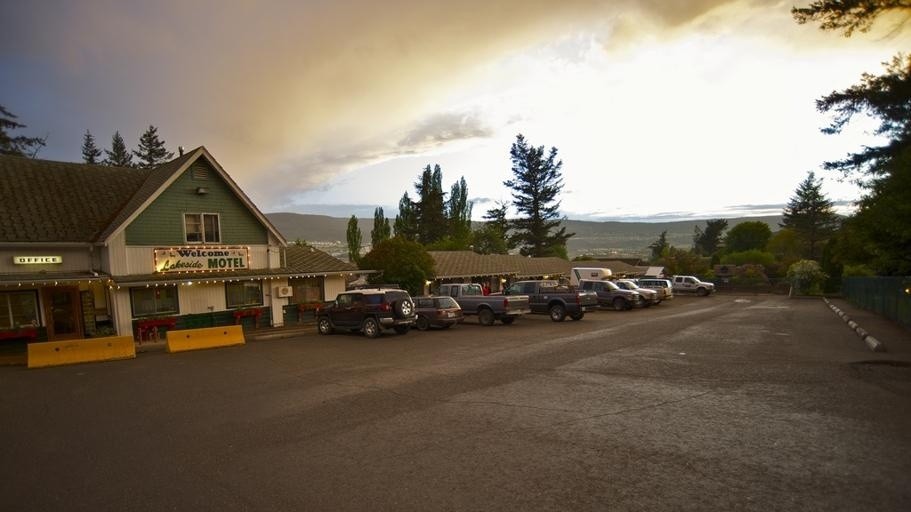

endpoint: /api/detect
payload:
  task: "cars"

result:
[408,294,463,330]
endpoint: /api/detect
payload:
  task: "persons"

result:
[482,281,491,296]
[502,283,507,295]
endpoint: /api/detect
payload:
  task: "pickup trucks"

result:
[671,274,716,297]
[491,279,598,321]
[435,282,532,326]
[578,279,640,311]
[613,280,657,308]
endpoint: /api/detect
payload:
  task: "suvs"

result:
[317,283,418,338]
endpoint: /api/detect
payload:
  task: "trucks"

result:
[570,267,617,290]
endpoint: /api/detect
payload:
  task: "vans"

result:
[639,278,672,304]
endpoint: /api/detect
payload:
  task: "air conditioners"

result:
[277,286,293,297]
